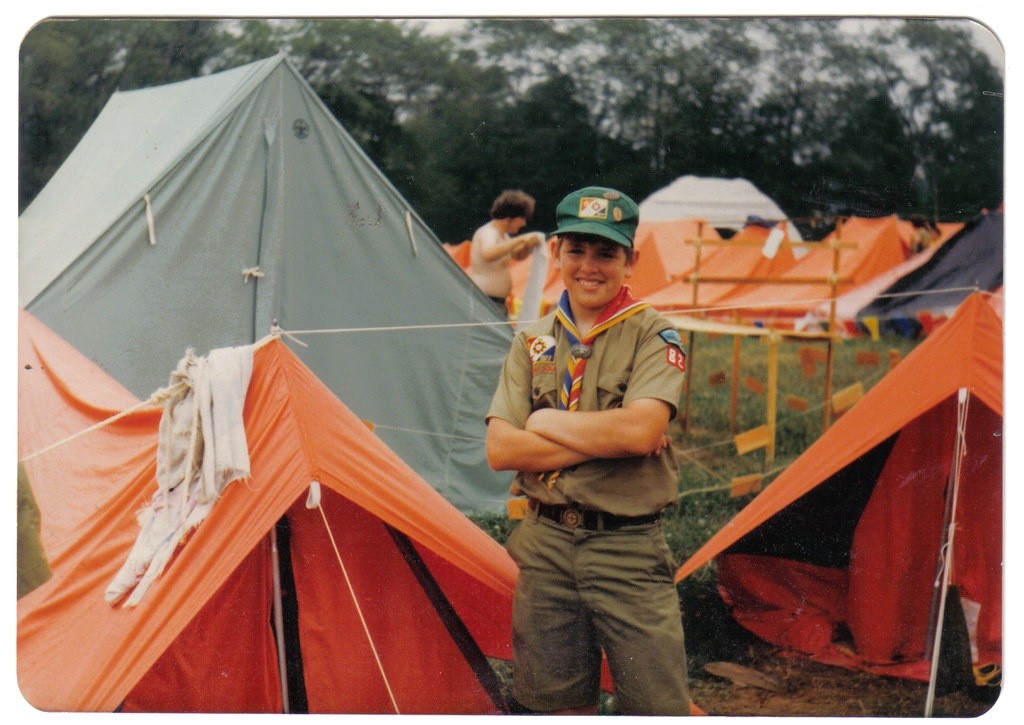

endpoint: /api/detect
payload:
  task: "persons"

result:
[469,191,540,316]
[484,186,689,716]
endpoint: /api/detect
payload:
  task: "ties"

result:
[538,283,653,489]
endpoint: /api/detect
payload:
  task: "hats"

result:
[549,187,640,248]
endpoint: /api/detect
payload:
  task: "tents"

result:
[673,289,1004,685]
[634,216,1003,342]
[18,53,530,517]
[639,174,804,242]
[17,307,705,716]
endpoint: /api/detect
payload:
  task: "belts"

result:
[490,297,505,304]
[527,499,657,531]
